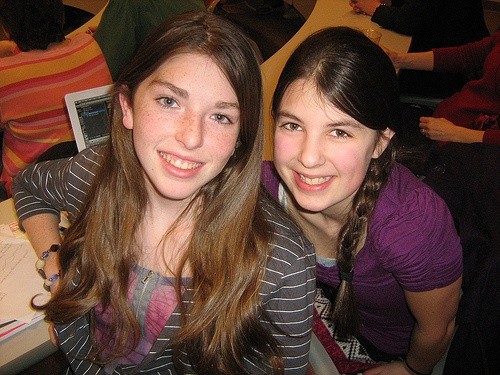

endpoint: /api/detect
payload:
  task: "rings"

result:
[421,128,425,135]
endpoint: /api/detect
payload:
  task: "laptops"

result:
[64,83,113,152]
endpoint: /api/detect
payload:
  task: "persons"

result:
[11,10,316,375]
[348,0,500,143]
[0,0,206,206]
[261,26,463,375]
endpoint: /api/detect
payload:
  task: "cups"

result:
[361,29,383,46]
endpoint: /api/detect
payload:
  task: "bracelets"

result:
[404,358,424,375]
[36,243,62,291]
[380,4,385,6]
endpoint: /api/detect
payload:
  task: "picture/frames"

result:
[65,84,115,153]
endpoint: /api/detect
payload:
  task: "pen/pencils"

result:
[58,225,69,235]
